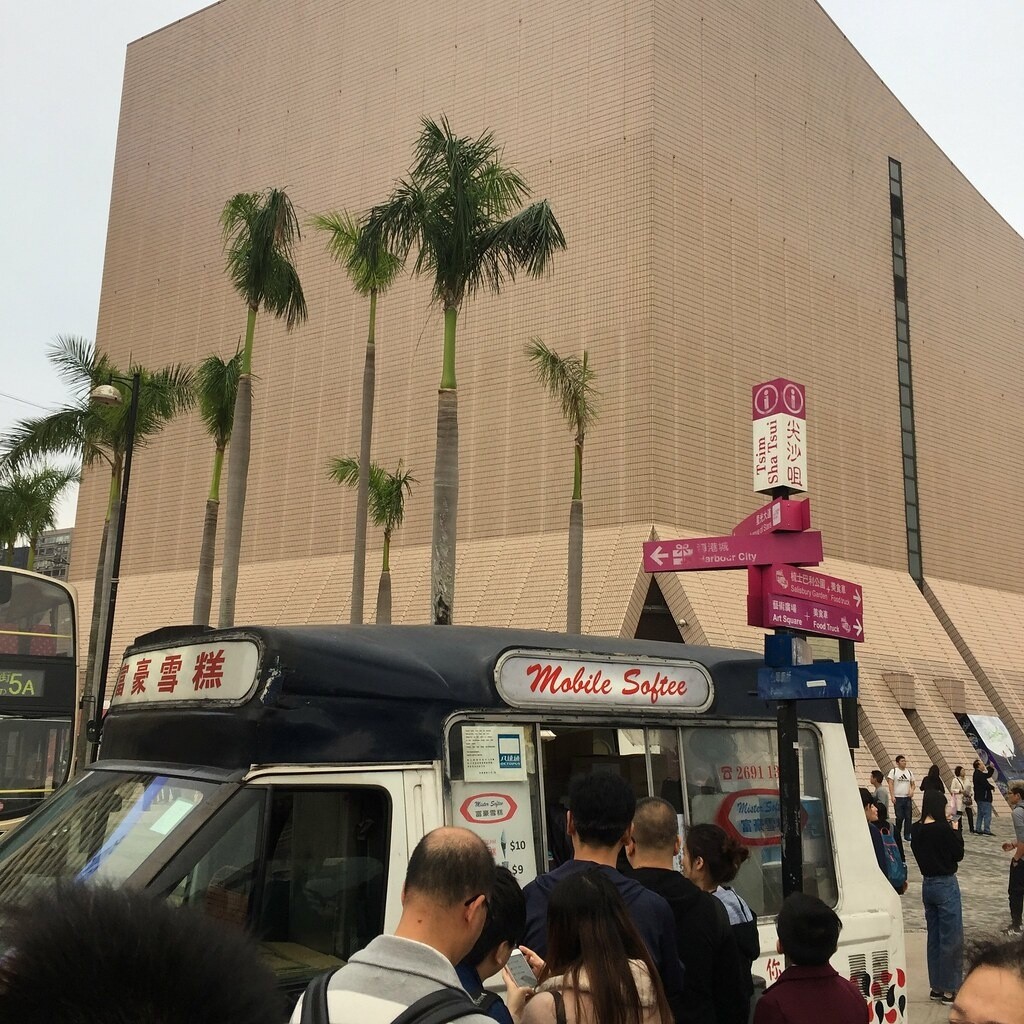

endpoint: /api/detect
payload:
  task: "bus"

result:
[1,565,99,876]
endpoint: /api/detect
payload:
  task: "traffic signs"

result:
[768,595,864,642]
[732,497,782,535]
[772,562,863,614]
[643,530,823,572]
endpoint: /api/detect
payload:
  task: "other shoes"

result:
[930,989,944,1000]
[983,832,996,836]
[903,836,912,842]
[975,831,983,835]
[941,992,957,1004]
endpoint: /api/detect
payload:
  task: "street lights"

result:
[79,372,141,854]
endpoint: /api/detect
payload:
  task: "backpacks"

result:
[880,823,908,887]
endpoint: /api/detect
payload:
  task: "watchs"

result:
[1012,858,1018,862]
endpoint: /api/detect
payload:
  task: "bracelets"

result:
[1011,843,1015,849]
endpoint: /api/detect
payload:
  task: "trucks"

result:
[0,624,909,1024]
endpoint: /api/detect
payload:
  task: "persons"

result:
[951,766,979,835]
[920,765,945,800]
[504,772,760,1024]
[910,793,964,1005]
[888,756,915,841]
[859,787,908,894]
[870,771,890,809]
[754,894,869,1024]
[0,882,278,1024]
[1002,782,1024,936]
[948,931,1024,1024]
[973,760,997,837]
[290,827,526,1024]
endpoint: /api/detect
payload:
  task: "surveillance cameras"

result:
[679,619,686,627]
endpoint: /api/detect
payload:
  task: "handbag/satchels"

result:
[950,792,957,817]
[963,795,973,805]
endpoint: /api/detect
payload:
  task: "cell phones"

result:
[504,949,539,999]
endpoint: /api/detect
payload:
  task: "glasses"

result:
[465,894,494,928]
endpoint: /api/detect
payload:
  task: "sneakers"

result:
[999,927,1023,936]
